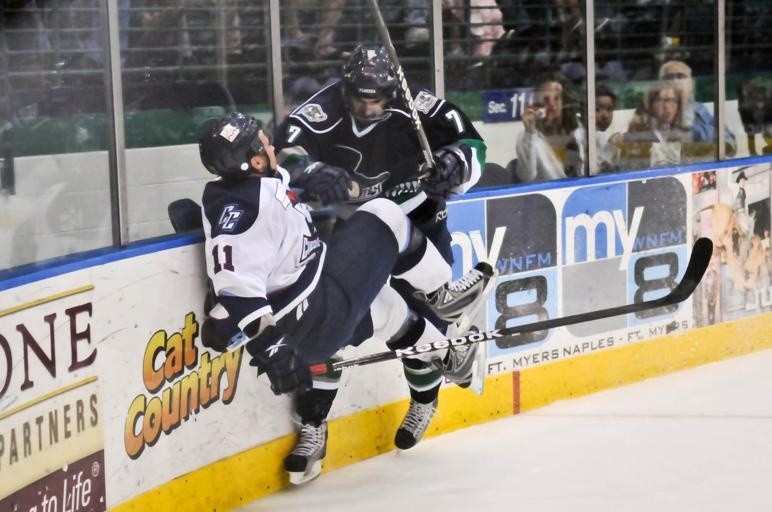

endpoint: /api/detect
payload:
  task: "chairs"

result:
[168,198,203,234]
[481,158,519,188]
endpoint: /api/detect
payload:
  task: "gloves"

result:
[290,159,353,207]
[244,325,313,396]
[418,142,473,197]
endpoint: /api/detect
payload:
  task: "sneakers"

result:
[284,419,328,472]
[393,394,440,450]
[411,261,493,325]
[431,324,480,389]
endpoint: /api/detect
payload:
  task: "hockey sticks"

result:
[309,238,713,377]
[174,83,238,115]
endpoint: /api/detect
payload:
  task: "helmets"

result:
[198,111,263,178]
[339,42,398,99]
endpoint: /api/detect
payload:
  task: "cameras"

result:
[533,105,547,119]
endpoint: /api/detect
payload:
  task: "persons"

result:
[692,168,771,327]
[256,40,490,474]
[0,1,348,118]
[193,110,493,398]
[400,0,772,184]
